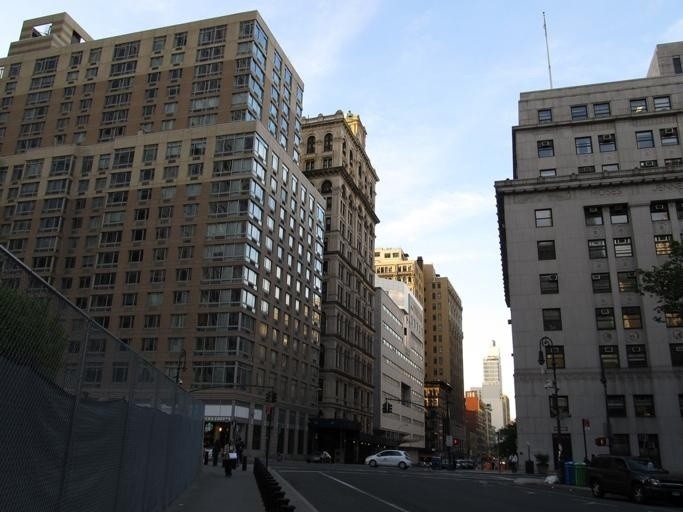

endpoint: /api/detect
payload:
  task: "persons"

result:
[213,435,248,478]
[509,451,520,473]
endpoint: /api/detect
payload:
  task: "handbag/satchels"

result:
[229,453,237,459]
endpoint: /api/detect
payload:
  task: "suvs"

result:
[585,452,682,505]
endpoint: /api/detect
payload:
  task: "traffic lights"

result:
[265,391,270,403]
[271,391,278,402]
[388,403,392,412]
[382,402,387,412]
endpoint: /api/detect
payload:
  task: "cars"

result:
[365,450,412,470]
[419,456,488,471]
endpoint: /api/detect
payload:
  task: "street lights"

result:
[171,348,189,415]
[535,333,563,484]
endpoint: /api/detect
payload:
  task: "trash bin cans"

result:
[574,461,587,486]
[563,462,575,484]
